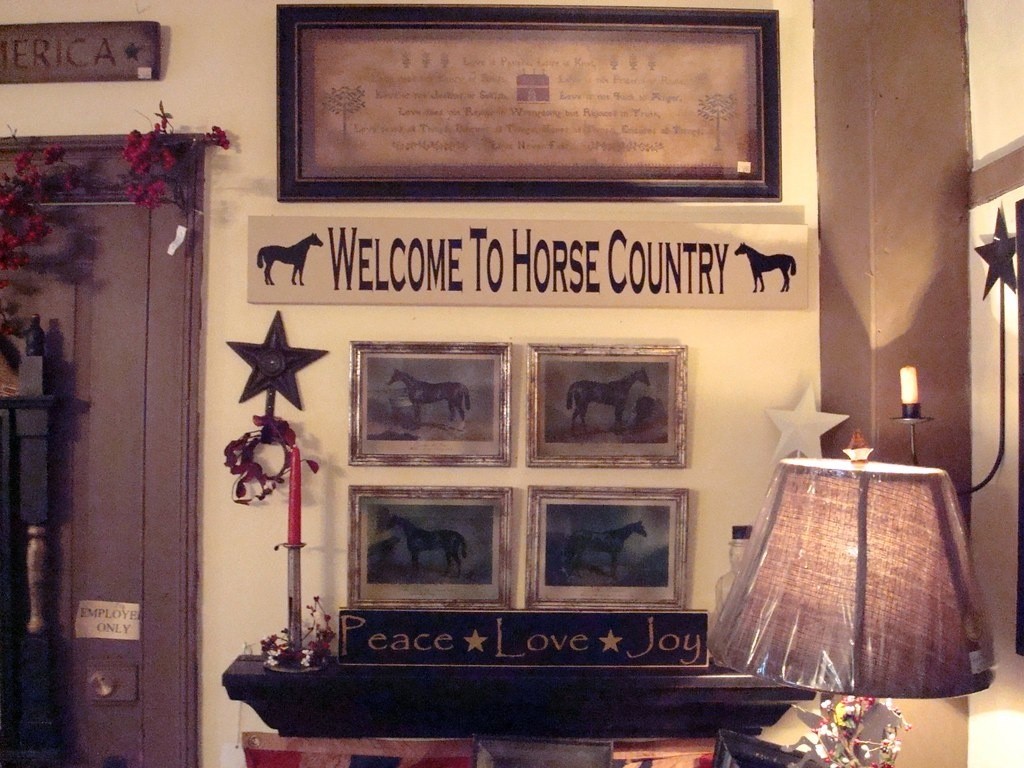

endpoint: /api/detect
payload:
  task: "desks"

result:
[222,654,816,768]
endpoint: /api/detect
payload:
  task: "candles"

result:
[287,446,308,544]
[900,365,919,403]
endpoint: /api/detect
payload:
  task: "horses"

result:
[385,516,467,579]
[386,368,471,431]
[566,367,651,431]
[563,520,647,579]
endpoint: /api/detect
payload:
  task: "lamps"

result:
[705,451,1000,768]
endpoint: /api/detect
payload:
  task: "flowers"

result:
[0,100,233,376]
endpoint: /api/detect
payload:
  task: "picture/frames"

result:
[525,343,687,470]
[524,485,690,610]
[276,2,781,200]
[347,339,513,468]
[345,483,513,611]
